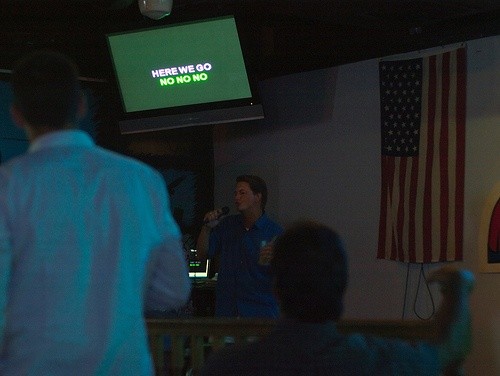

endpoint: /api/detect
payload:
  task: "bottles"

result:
[258,240,270,266]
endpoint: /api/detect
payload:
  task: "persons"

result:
[0,44,192,376]
[192,175,287,323]
[197,220,435,376]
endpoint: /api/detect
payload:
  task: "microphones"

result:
[200,207,229,224]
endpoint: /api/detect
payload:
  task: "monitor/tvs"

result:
[99,6,266,136]
[184,241,210,283]
